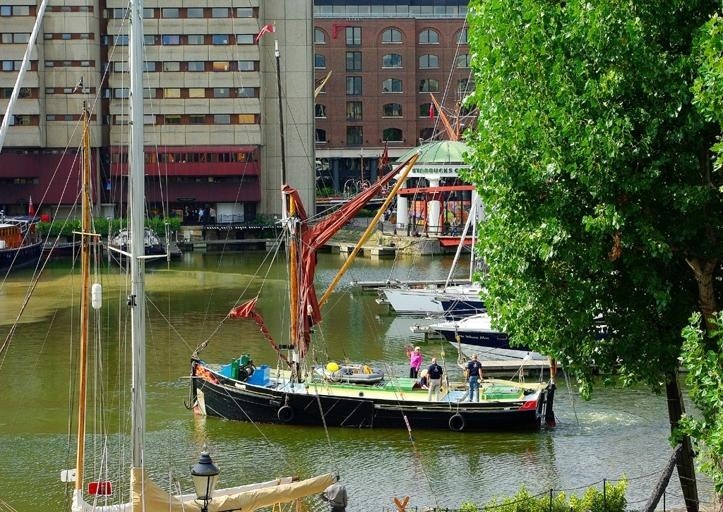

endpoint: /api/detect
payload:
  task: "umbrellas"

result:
[27,195,35,216]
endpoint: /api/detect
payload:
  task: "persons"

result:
[412,368,429,390]
[144,204,216,228]
[406,346,422,379]
[319,474,347,511]
[426,357,443,403]
[465,354,483,403]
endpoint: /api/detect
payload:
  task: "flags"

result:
[72,75,84,94]
[254,23,276,44]
[377,141,389,169]
